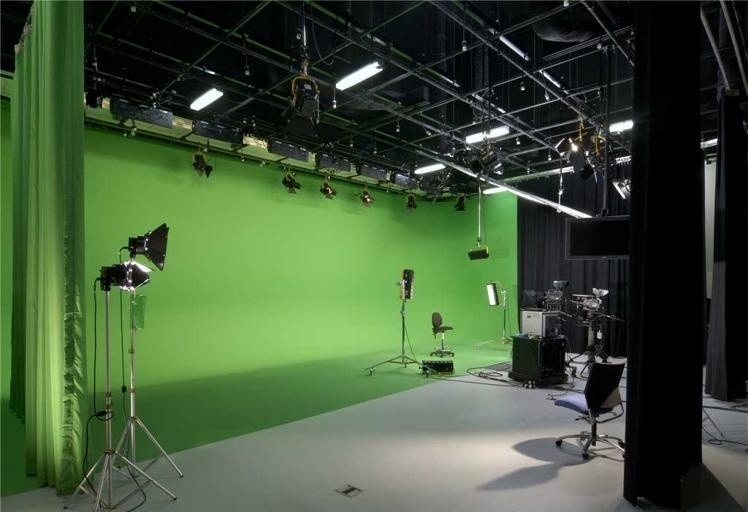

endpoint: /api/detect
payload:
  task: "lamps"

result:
[100,224,183,480]
[314,177,340,202]
[335,62,387,94]
[279,173,304,199]
[190,84,226,113]
[355,187,376,210]
[555,123,587,158]
[611,178,633,201]
[474,280,514,350]
[401,193,422,212]
[610,118,633,136]
[193,151,218,180]
[450,192,470,211]
[464,125,512,146]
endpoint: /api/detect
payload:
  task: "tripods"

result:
[103,257,184,487]
[474,290,513,350]
[364,279,440,378]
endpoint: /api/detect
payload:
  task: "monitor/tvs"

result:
[564,215,632,262]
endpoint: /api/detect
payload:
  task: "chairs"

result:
[432,313,455,357]
[552,363,627,460]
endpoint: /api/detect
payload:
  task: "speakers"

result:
[512,335,565,378]
[469,250,489,260]
